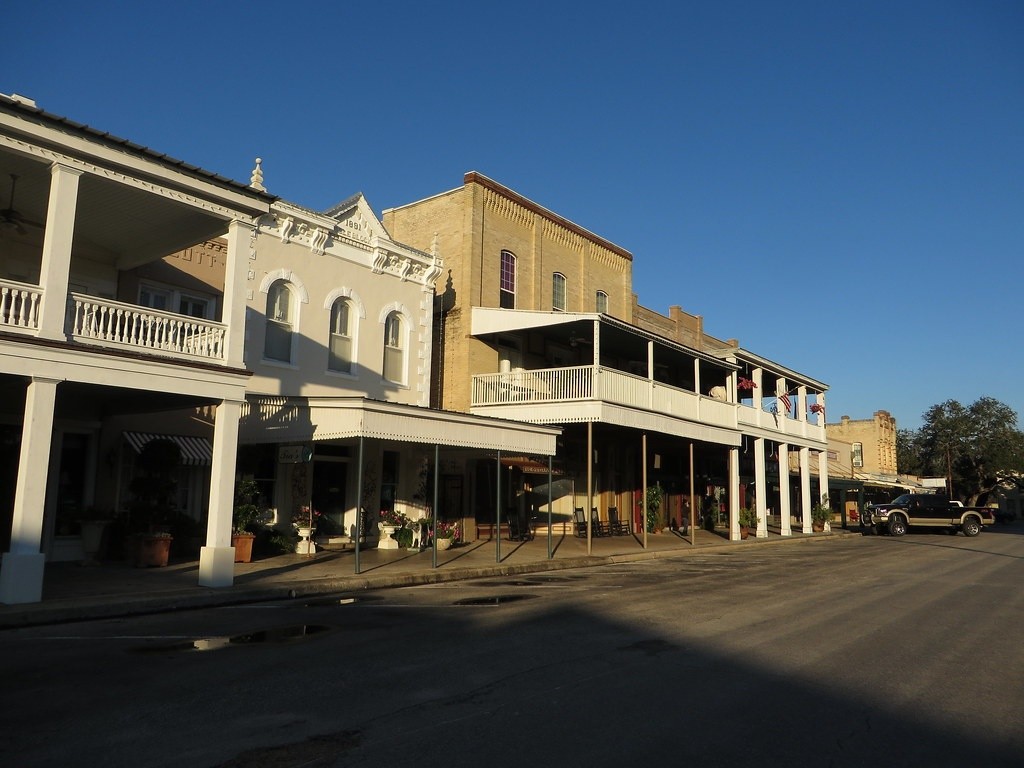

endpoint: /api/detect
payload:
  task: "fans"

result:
[0,173,44,235]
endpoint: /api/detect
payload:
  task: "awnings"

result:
[122,430,212,465]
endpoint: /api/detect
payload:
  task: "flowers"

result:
[379,510,403,525]
[291,505,322,527]
[429,520,459,539]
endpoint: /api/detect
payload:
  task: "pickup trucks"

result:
[861,494,1000,537]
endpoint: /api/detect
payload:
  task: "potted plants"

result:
[80,503,115,552]
[125,501,173,567]
[739,507,757,539]
[811,493,836,532]
[232,479,274,562]
[653,516,664,534]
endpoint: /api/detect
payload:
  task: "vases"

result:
[377,523,402,549]
[432,538,451,551]
[295,527,318,553]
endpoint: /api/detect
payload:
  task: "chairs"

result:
[591,507,612,537]
[608,507,631,536]
[849,509,859,522]
[573,507,587,537]
[505,506,532,541]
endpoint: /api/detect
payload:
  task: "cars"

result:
[949,501,963,507]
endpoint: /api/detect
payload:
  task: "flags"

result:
[779,394,792,412]
